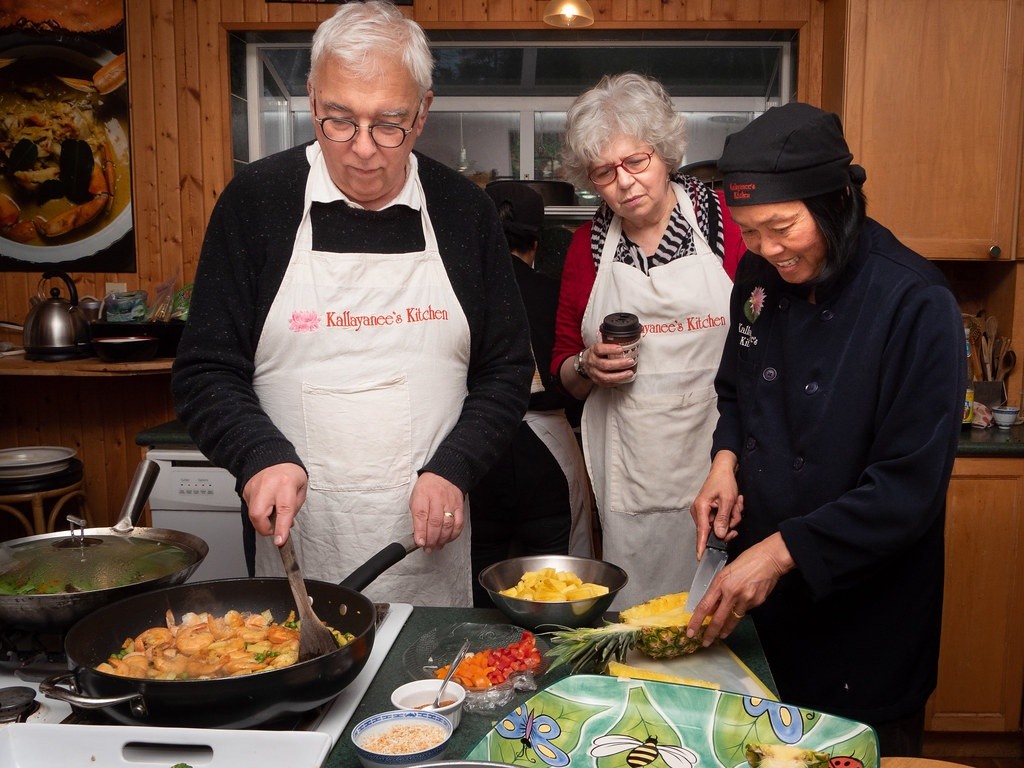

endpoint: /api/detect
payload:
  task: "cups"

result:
[599,313,642,385]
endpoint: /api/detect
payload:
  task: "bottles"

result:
[961,329,973,434]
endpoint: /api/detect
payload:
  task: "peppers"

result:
[435,631,540,687]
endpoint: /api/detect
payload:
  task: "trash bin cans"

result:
[144,449,244,586]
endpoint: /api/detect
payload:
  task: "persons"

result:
[549,73,749,611]
[689,102,968,757]
[472,198,595,577]
[170,0,536,608]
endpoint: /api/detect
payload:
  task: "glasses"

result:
[315,83,425,149]
[589,149,656,186]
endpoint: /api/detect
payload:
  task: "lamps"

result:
[543,0,594,28]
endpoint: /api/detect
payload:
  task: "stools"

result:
[0,458,94,536]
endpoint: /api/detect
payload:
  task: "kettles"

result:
[22,269,90,362]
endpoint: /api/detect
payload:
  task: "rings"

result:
[444,512,454,517]
[732,610,745,619]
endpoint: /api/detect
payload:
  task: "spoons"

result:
[420,641,471,710]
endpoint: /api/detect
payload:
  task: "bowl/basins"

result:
[403,760,531,768]
[478,555,629,632]
[85,320,185,358]
[484,180,575,207]
[350,709,453,767]
[0,446,77,479]
[0,38,133,263]
[391,678,465,731]
[991,406,1020,430]
[91,335,160,364]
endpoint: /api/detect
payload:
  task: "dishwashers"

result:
[145,448,249,584]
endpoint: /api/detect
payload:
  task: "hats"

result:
[717,106,866,206]
[487,184,544,229]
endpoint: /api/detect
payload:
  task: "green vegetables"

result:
[3,138,93,204]
[0,548,146,595]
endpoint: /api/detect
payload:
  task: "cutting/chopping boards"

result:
[605,638,780,703]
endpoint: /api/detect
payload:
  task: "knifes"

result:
[681,526,729,613]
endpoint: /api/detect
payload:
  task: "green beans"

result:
[255,620,350,662]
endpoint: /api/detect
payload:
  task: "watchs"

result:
[574,351,590,380]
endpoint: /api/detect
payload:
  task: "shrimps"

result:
[95,609,358,680]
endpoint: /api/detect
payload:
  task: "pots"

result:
[38,532,424,728]
[0,460,210,656]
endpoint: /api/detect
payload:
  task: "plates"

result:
[464,673,881,767]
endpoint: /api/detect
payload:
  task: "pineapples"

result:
[499,568,610,602]
[621,590,730,659]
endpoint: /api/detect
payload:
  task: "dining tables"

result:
[0,599,782,768]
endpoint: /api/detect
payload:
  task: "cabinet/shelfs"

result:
[840,0,1024,268]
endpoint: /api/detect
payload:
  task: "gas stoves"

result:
[0,601,412,768]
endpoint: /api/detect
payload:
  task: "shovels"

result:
[271,511,346,663]
[985,316,998,365]
